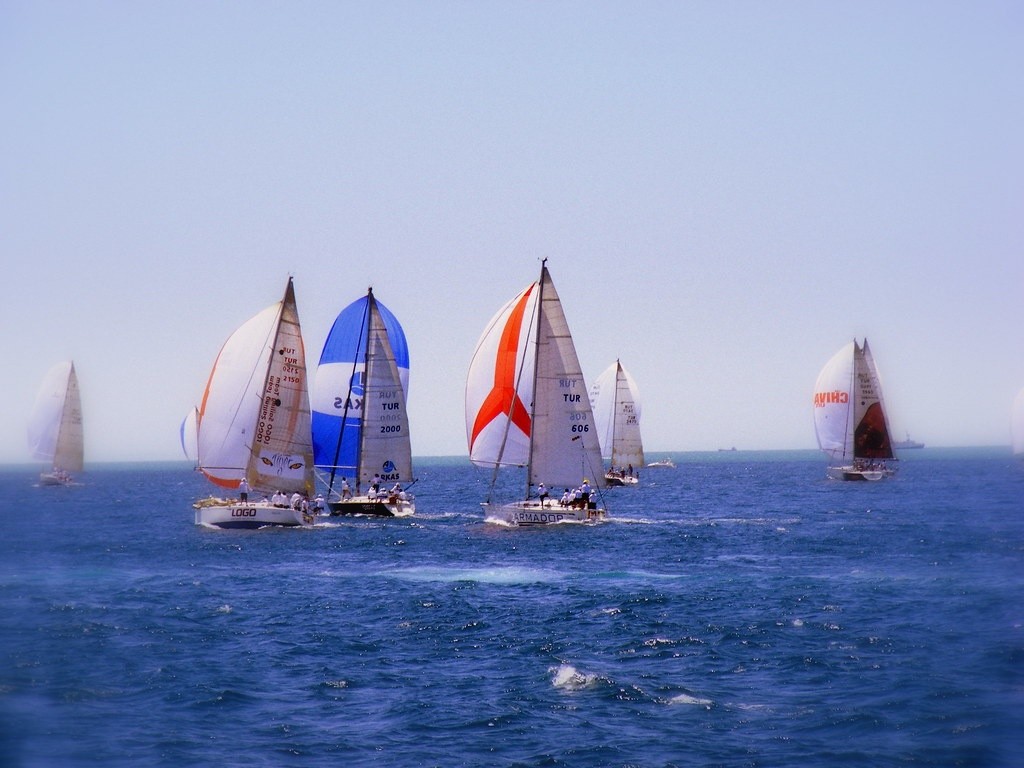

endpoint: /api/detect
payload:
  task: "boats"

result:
[647,461,675,468]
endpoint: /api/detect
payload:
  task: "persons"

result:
[238,478,251,506]
[852,459,887,472]
[341,477,353,501]
[620,467,625,479]
[368,487,376,502]
[399,489,407,499]
[560,481,597,509]
[271,490,309,514]
[313,494,324,516]
[635,472,639,478]
[368,474,382,493]
[390,483,400,492]
[609,466,614,478]
[538,483,551,509]
[627,464,633,476]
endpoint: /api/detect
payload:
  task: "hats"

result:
[578,487,581,490]
[396,482,400,487]
[539,483,543,486]
[241,478,247,482]
[591,489,595,493]
[318,494,322,497]
[572,489,575,492]
[583,480,588,483]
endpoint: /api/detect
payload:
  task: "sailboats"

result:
[465,256,613,526]
[814,336,903,480]
[24,358,87,485]
[310,285,422,518]
[585,358,645,487]
[178,276,316,529]
